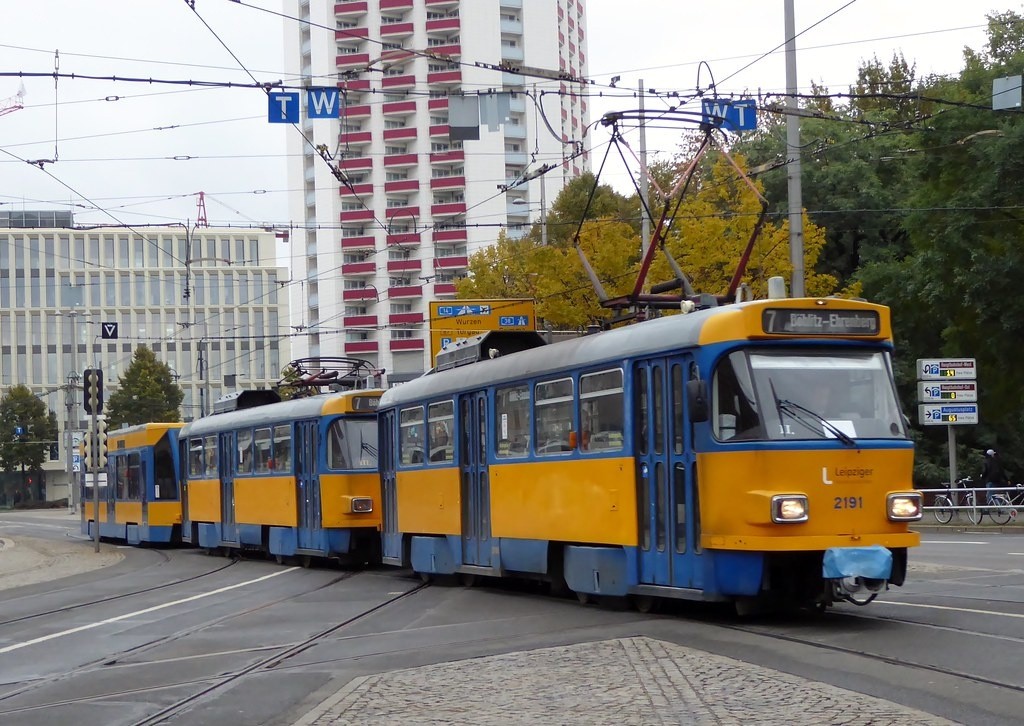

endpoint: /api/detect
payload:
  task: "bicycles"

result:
[987,483,1024,524]
[933,476,983,524]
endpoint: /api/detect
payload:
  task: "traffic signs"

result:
[916,358,977,380]
[917,404,979,425]
[917,381,978,403]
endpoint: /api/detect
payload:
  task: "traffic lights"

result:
[84,369,103,416]
[50,443,59,460]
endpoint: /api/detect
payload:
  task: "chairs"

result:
[187,429,624,475]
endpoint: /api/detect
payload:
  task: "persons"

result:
[581,409,604,442]
[13,490,21,506]
[412,434,433,463]
[436,431,448,461]
[981,449,1011,515]
[805,381,831,419]
[560,421,571,450]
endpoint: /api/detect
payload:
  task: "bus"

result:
[178,389,388,567]
[79,423,189,546]
[376,299,922,616]
